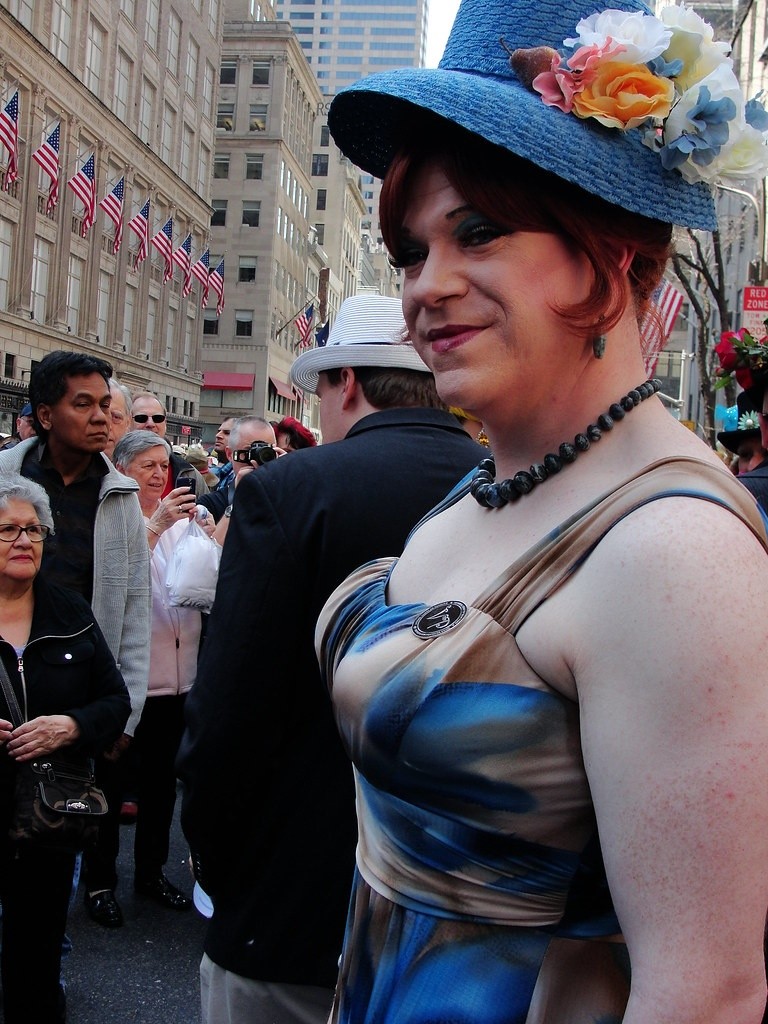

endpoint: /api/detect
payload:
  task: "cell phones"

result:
[175,478,196,513]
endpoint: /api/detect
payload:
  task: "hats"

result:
[20,402,33,417]
[185,450,220,487]
[172,445,183,454]
[327,0,768,230]
[718,392,764,454]
[290,295,432,393]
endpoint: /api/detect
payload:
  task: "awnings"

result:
[269,376,296,401]
[293,386,310,403]
[203,372,255,392]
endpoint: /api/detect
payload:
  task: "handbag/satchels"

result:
[8,758,109,848]
[165,512,223,614]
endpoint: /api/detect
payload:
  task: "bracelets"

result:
[145,525,160,537]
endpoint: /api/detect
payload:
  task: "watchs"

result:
[224,503,233,517]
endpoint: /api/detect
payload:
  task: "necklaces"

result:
[468,372,661,507]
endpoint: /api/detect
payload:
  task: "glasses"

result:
[21,417,34,426]
[0,523,50,542]
[132,415,165,423]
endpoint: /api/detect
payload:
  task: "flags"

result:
[98,176,125,256]
[315,319,330,347]
[209,259,226,316]
[191,249,212,308]
[293,302,314,348]
[172,233,191,298]
[32,120,61,215]
[151,216,173,284]
[638,273,682,383]
[68,152,97,238]
[0,87,18,193]
[127,198,150,273]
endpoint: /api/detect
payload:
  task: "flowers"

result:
[709,326,768,391]
[530,2,768,184]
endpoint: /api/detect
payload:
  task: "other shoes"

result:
[120,802,138,817]
[61,936,73,952]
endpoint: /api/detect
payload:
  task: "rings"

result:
[178,505,182,513]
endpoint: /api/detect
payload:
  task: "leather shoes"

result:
[136,875,193,913]
[84,890,124,928]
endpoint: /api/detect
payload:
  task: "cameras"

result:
[234,440,277,467]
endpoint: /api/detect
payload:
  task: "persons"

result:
[0,349,318,1024]
[182,285,495,1024]
[314,2,768,1024]
